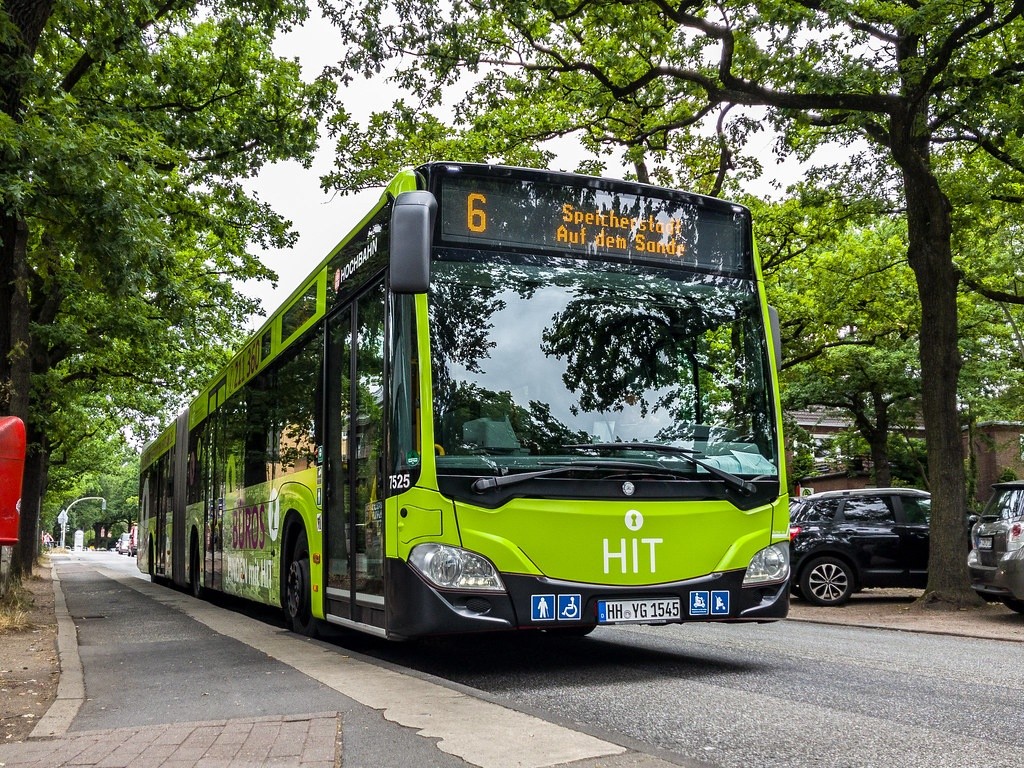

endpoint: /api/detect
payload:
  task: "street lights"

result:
[57,497,106,553]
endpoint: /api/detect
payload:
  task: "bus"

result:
[135,160,791,643]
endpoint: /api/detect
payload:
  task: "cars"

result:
[966,480,1023,618]
[788,488,980,608]
[114,524,140,558]
